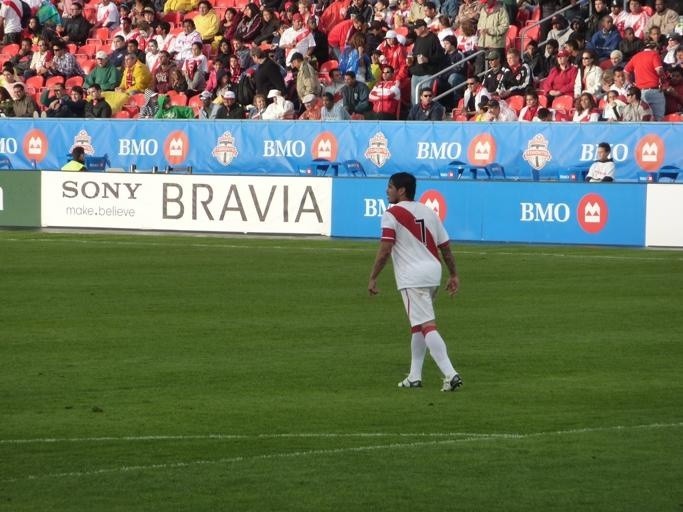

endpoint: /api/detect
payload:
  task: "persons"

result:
[366,171,463,392]
[0,0,682,120]
[581,141,616,183]
[58,146,87,172]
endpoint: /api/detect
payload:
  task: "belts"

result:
[640,87,658,90]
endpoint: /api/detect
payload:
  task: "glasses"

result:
[423,94,432,97]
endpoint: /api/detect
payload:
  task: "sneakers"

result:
[440,372,463,391]
[398,377,421,388]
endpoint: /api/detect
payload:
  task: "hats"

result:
[199,91,233,99]
[95,51,108,59]
[302,94,315,104]
[646,33,679,49]
[484,100,498,107]
[267,89,281,99]
[550,15,585,30]
[293,13,302,21]
[609,0,621,7]
[290,52,303,62]
[384,19,426,38]
[485,51,498,60]
[150,93,159,98]
[141,6,155,14]
[558,50,568,57]
